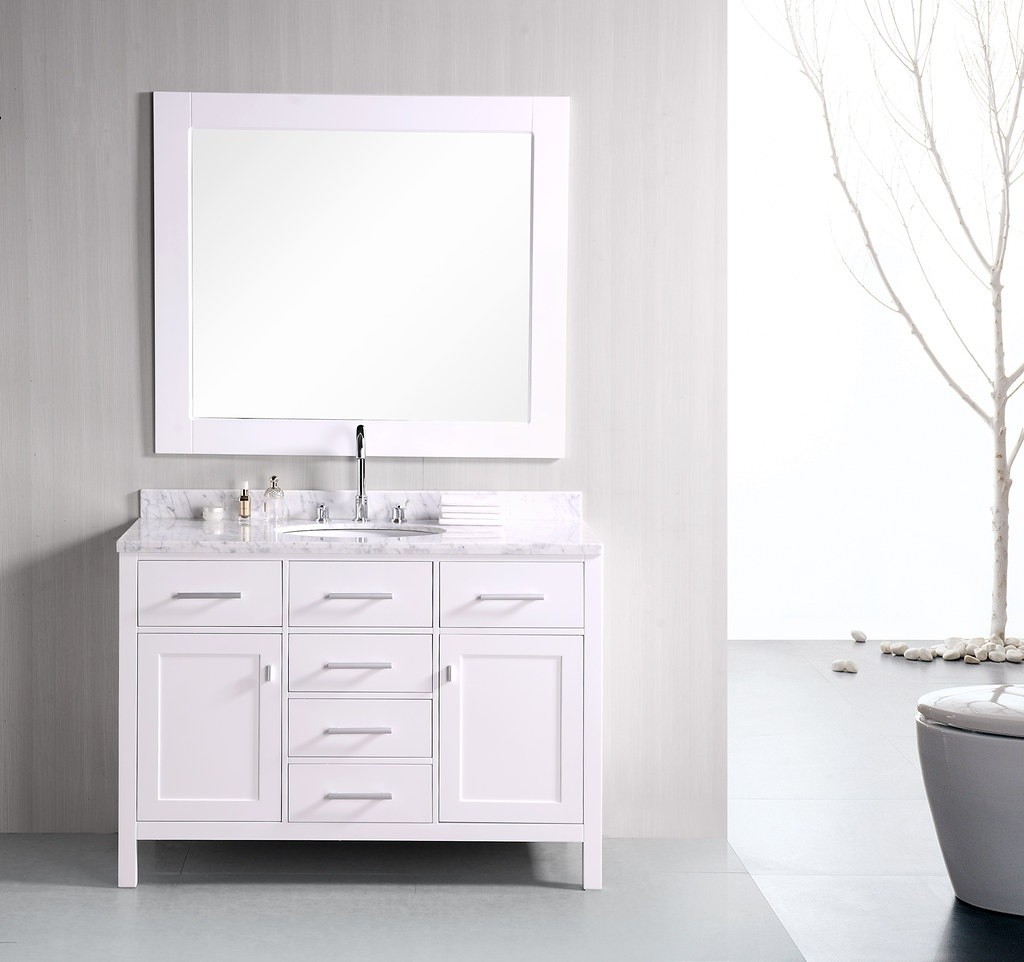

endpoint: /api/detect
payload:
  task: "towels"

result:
[439,495,502,527]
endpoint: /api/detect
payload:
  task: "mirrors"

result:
[152,88,566,460]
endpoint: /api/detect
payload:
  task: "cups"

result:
[202,506,224,520]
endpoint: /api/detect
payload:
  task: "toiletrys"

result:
[263,475,285,520]
[238,480,251,524]
[202,506,224,523]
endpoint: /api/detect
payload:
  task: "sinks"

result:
[273,521,449,541]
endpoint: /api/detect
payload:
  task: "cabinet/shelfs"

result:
[287,560,437,844]
[440,556,605,890]
[119,558,284,888]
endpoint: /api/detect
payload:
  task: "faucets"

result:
[352,424,371,523]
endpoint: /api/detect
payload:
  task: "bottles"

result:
[239,479,252,525]
[263,475,286,522]
